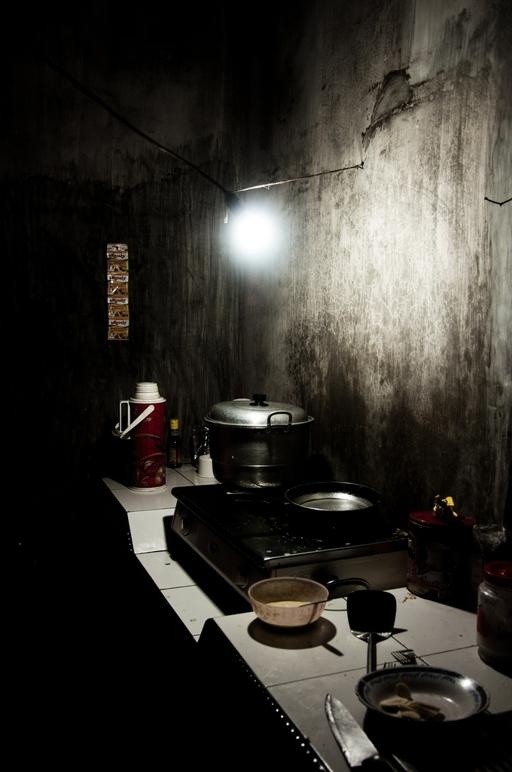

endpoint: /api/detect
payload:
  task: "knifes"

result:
[321,690,392,772]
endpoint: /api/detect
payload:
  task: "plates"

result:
[284,481,382,527]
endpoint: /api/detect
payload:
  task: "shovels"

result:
[347,589,396,675]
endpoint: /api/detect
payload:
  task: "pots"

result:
[201,391,314,492]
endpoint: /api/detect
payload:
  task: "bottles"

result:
[168,419,183,468]
[113,380,167,489]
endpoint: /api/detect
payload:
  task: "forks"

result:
[391,648,419,666]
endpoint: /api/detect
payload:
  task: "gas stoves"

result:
[170,478,412,607]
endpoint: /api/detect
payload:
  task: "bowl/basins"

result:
[244,574,330,631]
[353,664,489,724]
